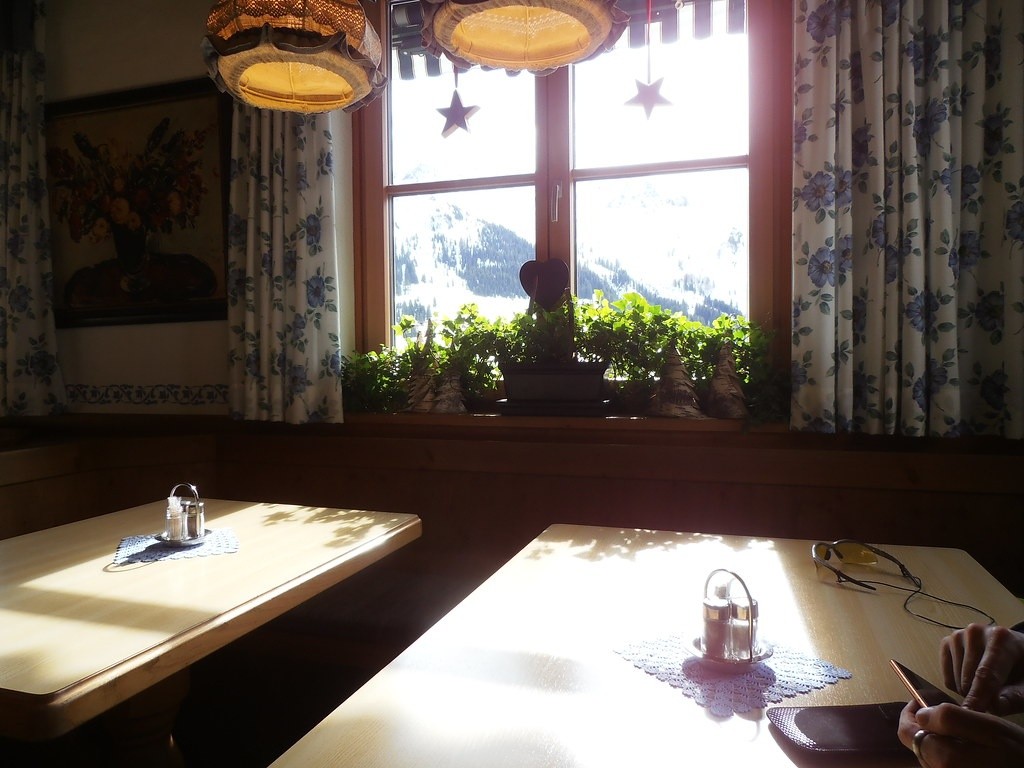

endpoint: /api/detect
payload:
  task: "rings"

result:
[913,730,934,759]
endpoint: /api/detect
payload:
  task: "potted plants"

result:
[496,307,613,417]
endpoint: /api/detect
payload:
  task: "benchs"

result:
[0,416,1024,699]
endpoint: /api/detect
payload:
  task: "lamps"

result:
[419,0,631,77]
[198,0,389,116]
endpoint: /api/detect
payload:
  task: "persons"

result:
[897,623,1024,768]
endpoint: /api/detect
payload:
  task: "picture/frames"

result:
[44,72,236,328]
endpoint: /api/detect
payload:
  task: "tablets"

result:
[889,659,961,709]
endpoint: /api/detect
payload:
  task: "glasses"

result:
[811,539,922,591]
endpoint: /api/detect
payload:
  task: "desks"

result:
[269,524,1024,768]
[0,496,422,768]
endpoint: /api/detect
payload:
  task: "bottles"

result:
[702,598,730,655]
[730,598,759,657]
[187,502,205,536]
[180,501,191,514]
[166,496,183,540]
[713,584,732,602]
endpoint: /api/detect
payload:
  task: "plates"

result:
[154,529,212,546]
[684,636,774,673]
